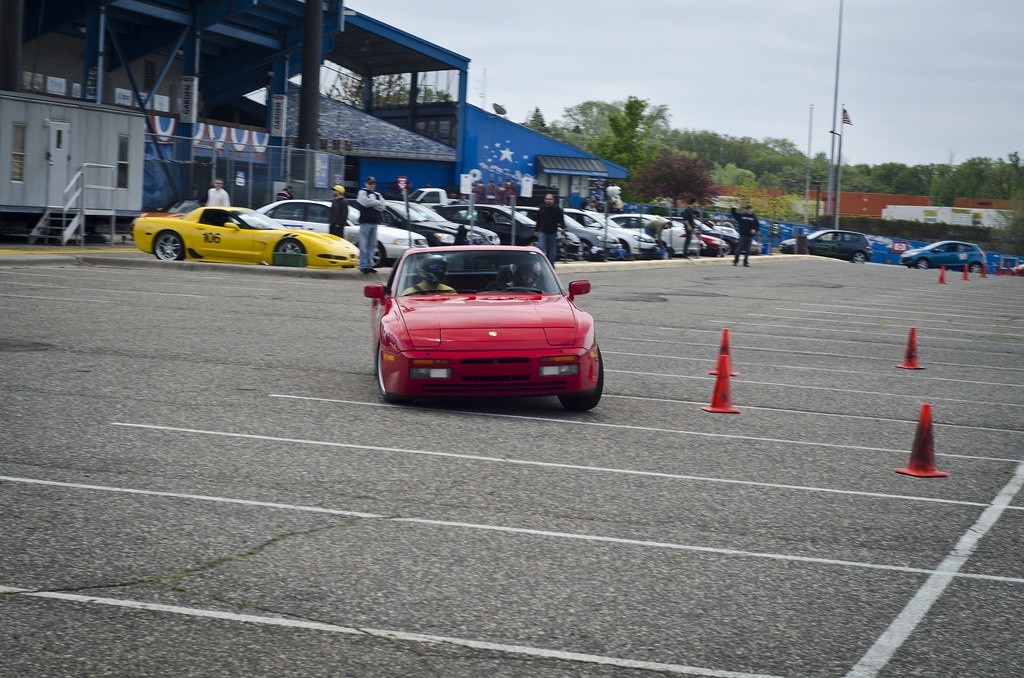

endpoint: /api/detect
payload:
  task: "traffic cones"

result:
[893,405,950,478]
[938,262,1020,286]
[708,327,739,376]
[702,354,741,416]
[895,327,924,370]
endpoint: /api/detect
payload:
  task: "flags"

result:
[841,109,853,125]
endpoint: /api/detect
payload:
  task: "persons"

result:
[534,194,565,270]
[731,204,760,267]
[486,264,541,292]
[681,199,698,259]
[644,218,673,259]
[211,212,241,231]
[276,186,294,201]
[356,176,386,274]
[403,255,456,295]
[329,184,351,238]
[204,178,230,206]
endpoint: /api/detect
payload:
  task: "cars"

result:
[780,227,873,265]
[128,192,760,273]
[362,244,607,412]
[899,240,987,273]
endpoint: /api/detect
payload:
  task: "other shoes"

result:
[361,268,377,273]
[744,263,750,267]
[683,255,691,259]
[733,263,737,266]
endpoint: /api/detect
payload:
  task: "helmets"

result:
[421,255,449,284]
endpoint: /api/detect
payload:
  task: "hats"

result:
[330,185,345,194]
[364,176,376,183]
[743,205,752,210]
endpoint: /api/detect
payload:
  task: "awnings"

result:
[536,154,608,179]
